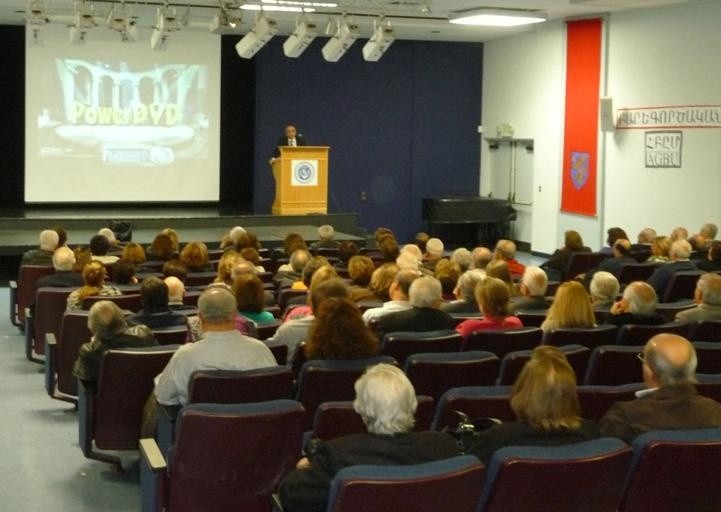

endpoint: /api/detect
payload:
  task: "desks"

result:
[422,195,511,250]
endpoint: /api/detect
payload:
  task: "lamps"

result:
[26,0,547,62]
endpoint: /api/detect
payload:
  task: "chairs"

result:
[311,390,437,443]
[326,453,490,511]
[71,345,183,473]
[11,247,721,430]
[622,429,720,509]
[488,434,637,511]
[139,399,305,512]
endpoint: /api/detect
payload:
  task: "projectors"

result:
[207,16,242,34]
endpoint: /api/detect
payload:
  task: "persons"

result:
[455,222,721,462]
[271,123,306,163]
[20,220,465,512]
